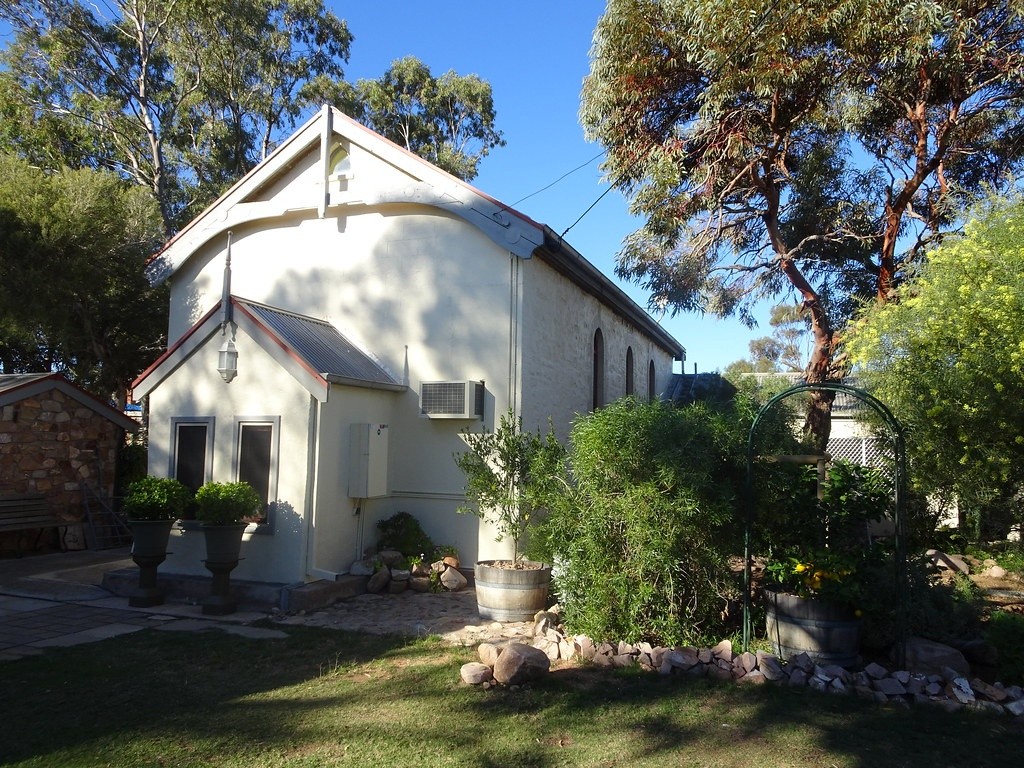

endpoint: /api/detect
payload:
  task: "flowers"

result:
[758,541,879,617]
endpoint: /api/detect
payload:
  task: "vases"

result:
[763,589,862,669]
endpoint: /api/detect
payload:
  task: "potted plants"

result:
[121,473,193,555]
[451,406,569,623]
[193,480,266,562]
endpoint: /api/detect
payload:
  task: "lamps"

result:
[217,339,238,384]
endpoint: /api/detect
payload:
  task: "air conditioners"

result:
[418,379,483,420]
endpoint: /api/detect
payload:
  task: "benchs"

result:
[0,494,73,559]
